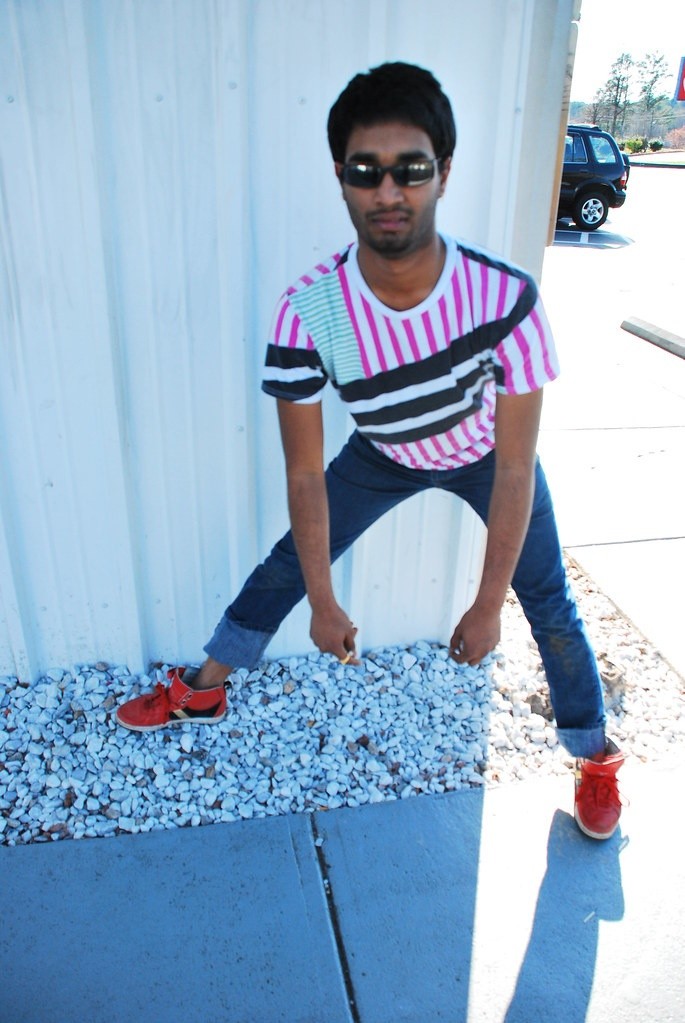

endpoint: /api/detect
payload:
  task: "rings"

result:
[340,655,350,664]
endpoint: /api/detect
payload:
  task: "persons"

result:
[117,64,625,840]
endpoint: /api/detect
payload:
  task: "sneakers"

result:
[574,735,625,839]
[115,666,227,732]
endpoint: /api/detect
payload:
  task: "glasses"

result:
[341,158,444,187]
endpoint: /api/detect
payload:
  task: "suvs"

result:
[554,125,630,231]
